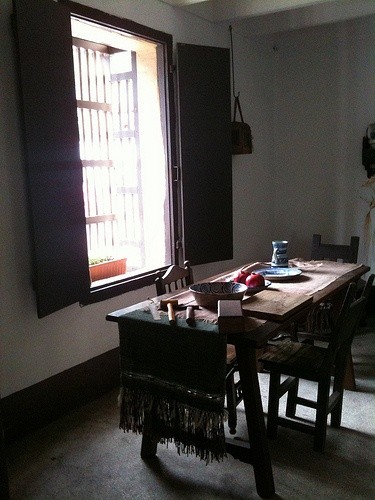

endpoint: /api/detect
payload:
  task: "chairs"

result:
[155,260,264,436]
[257,271,375,453]
[299,233,360,346]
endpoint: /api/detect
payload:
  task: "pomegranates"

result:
[238,271,265,289]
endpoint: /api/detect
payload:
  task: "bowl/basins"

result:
[229,279,271,296]
[189,282,247,308]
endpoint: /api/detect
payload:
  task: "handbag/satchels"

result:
[231,97,252,155]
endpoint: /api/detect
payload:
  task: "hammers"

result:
[160,300,178,323]
[179,304,199,323]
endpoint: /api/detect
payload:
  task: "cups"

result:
[271,240,288,268]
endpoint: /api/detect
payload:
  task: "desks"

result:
[106,258,371,496]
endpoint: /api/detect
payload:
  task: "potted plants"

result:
[89,255,127,283]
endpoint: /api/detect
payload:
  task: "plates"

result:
[252,268,303,281]
[297,260,321,271]
